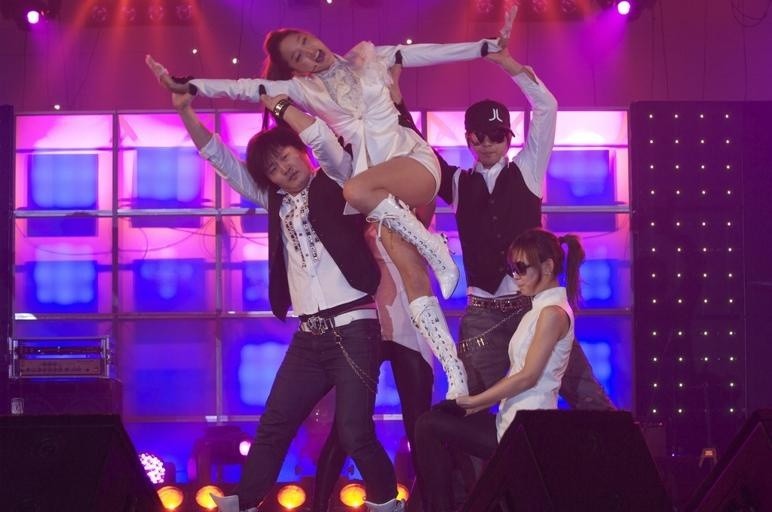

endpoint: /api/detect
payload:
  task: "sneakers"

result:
[208,493,257,512]
[363,498,406,512]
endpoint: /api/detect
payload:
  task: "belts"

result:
[297,309,378,335]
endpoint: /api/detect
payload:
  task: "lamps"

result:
[337,482,368,512]
[194,484,225,512]
[274,484,309,512]
[157,486,186,512]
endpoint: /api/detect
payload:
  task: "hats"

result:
[464,100,516,138]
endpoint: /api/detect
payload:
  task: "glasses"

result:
[467,129,505,145]
[503,255,555,278]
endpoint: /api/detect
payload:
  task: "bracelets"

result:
[272,99,292,120]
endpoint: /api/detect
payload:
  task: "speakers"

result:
[0,415,164,512]
[3,380,123,428]
[685,411,772,512]
[459,410,672,510]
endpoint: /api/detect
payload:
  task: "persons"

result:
[365,28,625,408]
[164,74,409,509]
[410,226,586,511]
[307,112,436,510]
[142,2,527,406]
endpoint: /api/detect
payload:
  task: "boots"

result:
[407,296,469,400]
[365,193,460,300]
